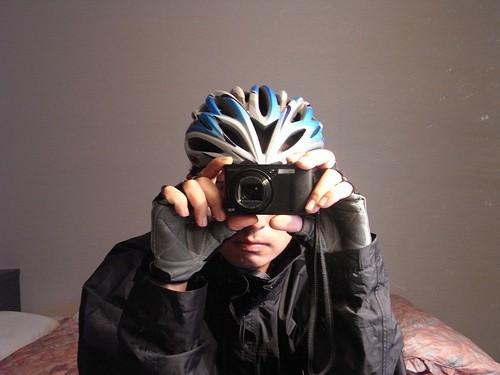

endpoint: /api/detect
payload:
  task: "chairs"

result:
[0,293,500,375]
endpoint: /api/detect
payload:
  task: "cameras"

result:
[223,163,314,215]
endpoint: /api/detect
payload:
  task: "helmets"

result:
[185,84,325,166]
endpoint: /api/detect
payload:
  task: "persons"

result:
[78,83,405,375]
